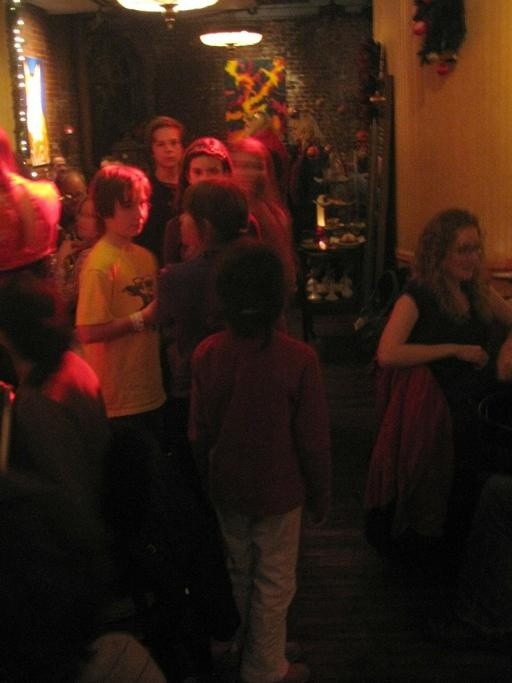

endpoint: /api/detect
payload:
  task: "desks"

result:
[298,234,368,343]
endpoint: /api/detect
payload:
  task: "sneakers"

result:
[226,639,309,683]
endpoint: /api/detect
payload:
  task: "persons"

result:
[361,209,512,637]
